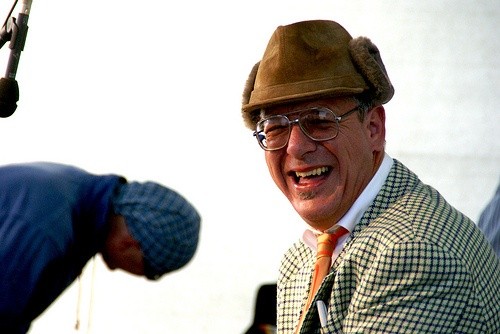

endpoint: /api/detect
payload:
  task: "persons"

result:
[0,162,201,334]
[243,20,500,334]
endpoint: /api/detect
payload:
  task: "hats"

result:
[240,19,396,130]
[115,181,200,276]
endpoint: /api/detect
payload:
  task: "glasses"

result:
[253,98,374,151]
[142,257,166,280]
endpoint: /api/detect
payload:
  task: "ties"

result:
[295,226,349,334]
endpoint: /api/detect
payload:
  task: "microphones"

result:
[0,0,32,117]
